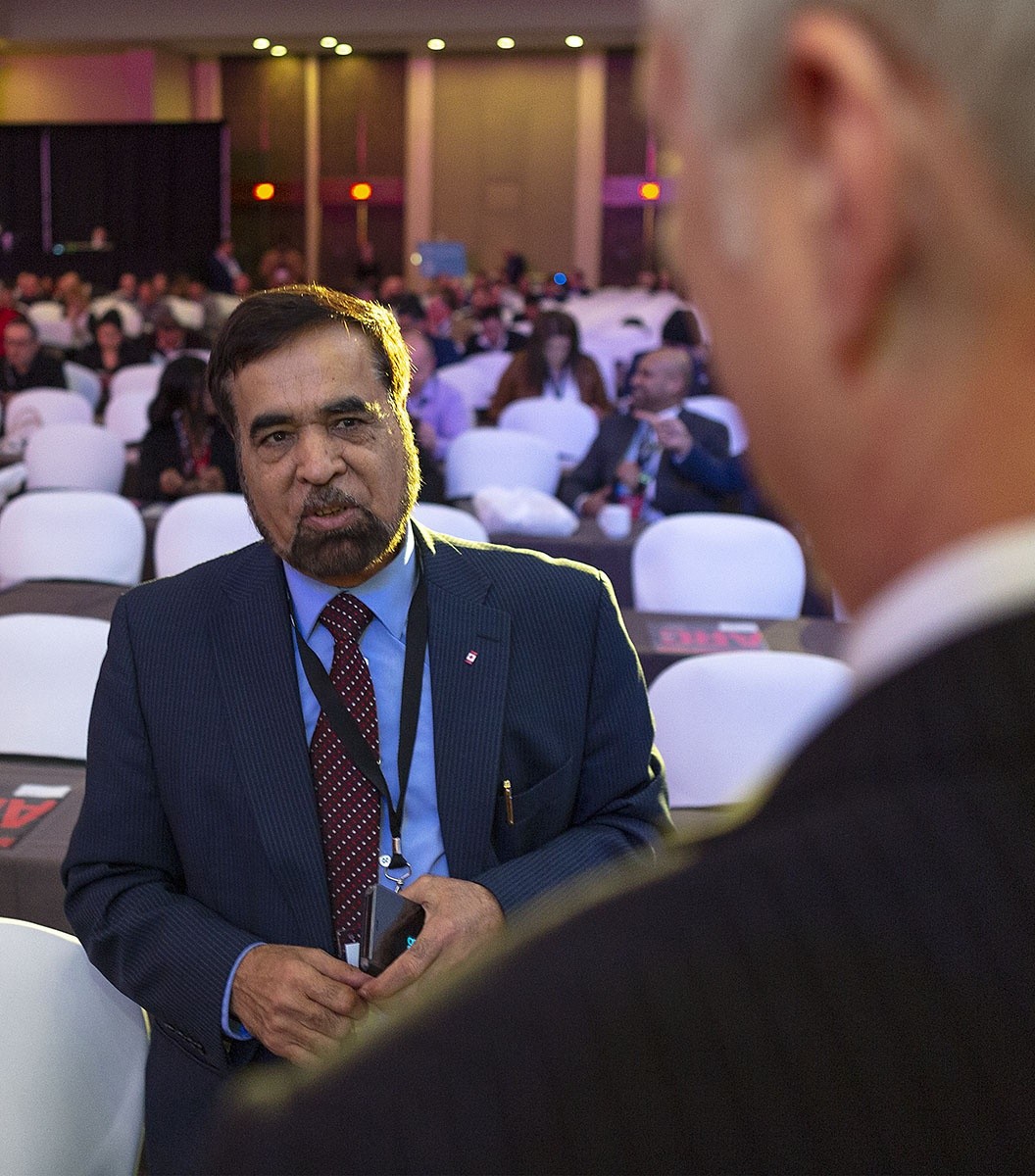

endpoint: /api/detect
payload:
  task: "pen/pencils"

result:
[502,780,514,823]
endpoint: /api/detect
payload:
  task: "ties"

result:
[309,592,380,965]
[635,425,652,468]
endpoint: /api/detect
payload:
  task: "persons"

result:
[0,227,751,521]
[242,0,1034,1176]
[61,289,677,1176]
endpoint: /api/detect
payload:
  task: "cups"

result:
[597,503,632,541]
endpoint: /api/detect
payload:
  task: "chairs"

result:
[0,292,867,1176]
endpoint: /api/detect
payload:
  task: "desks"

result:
[619,607,848,687]
[0,760,87,938]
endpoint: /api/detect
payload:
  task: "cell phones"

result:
[359,882,426,980]
